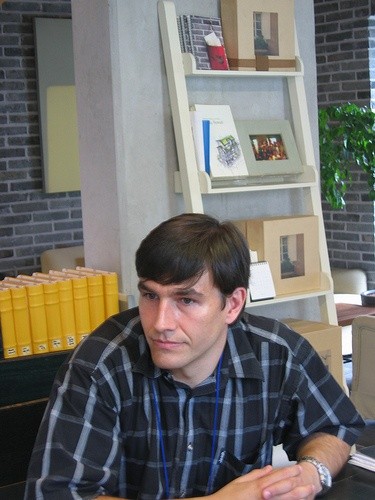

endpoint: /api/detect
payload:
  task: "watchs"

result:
[296,456,332,496]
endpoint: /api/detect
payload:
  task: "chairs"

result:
[40,244,85,273]
[348,317,375,418]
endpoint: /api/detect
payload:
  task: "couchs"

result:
[332,268,367,363]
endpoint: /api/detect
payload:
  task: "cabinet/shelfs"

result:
[158,0,350,399]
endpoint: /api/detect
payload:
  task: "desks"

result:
[335,302,374,327]
[312,463,375,500]
[0,349,73,500]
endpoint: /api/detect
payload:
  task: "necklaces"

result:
[150,352,224,500]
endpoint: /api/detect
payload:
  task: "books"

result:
[191,103,248,181]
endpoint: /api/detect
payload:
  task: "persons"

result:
[25,212,368,500]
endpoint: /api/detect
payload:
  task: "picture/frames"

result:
[234,119,301,176]
